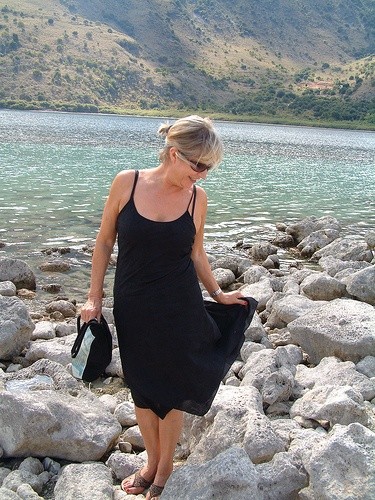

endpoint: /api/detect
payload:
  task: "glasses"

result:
[179,150,213,172]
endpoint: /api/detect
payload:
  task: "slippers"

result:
[146,482,164,500]
[121,466,153,495]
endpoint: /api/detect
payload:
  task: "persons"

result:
[81,115,259,500]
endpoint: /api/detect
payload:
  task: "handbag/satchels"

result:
[71,310,112,382]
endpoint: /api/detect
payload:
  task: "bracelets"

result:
[210,287,222,297]
